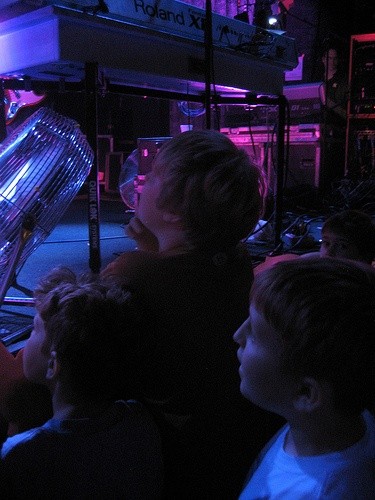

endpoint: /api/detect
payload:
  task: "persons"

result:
[0,132,262,433]
[232,254,375,500]
[318,212,375,265]
[0,267,164,499]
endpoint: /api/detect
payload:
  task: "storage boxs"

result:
[235,141,334,217]
